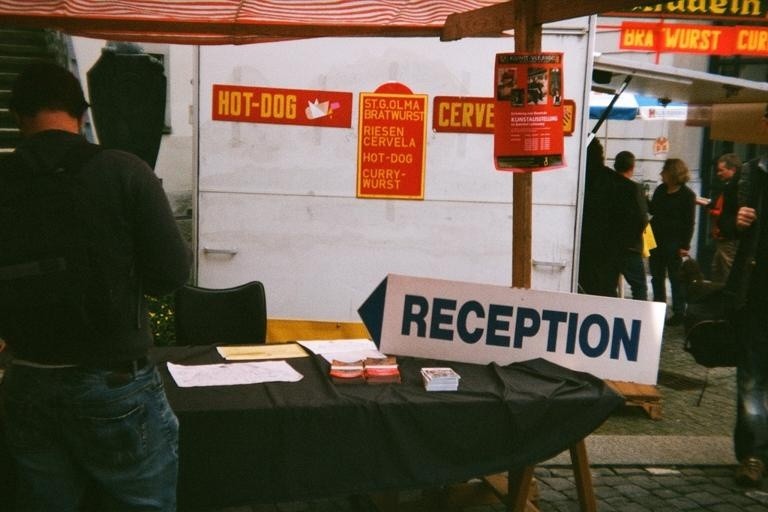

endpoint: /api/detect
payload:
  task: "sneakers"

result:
[665,313,692,324]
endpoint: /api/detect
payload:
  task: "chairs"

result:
[173,279,268,347]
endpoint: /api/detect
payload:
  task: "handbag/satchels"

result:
[679,282,742,367]
[674,251,705,302]
[641,219,658,259]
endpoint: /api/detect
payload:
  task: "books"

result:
[419,366,461,392]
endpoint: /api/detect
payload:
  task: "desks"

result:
[154,340,624,512]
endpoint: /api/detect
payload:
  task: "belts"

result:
[5,353,148,381]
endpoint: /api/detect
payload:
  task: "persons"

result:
[578,132,743,327]
[718,102,767,484]
[1,56,193,511]
[497,69,560,105]
